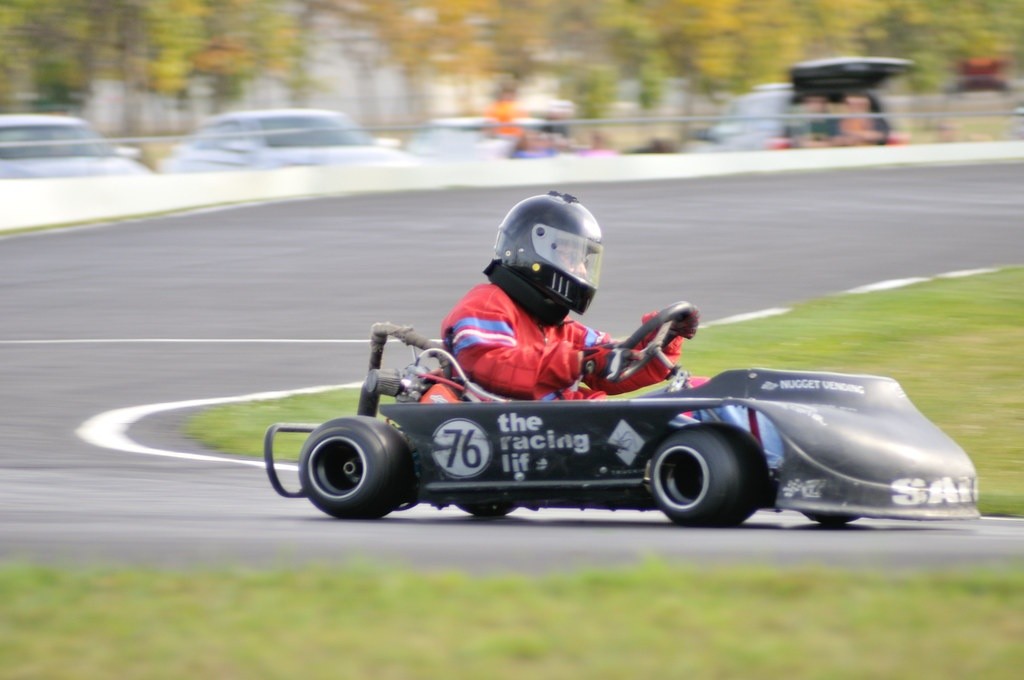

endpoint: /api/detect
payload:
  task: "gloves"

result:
[656,301,701,339]
[580,340,644,383]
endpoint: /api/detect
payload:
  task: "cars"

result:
[0,110,151,180]
[699,55,919,149]
[165,109,427,171]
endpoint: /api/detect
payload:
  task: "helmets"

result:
[492,190,604,315]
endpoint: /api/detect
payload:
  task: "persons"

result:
[491,83,571,152]
[783,91,888,150]
[440,190,698,403]
